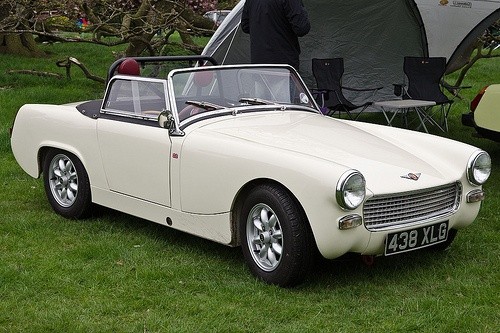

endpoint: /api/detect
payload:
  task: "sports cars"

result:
[9,55,492,289]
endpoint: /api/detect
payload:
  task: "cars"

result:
[202,10,232,28]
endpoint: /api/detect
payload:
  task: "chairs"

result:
[308,58,382,121]
[392,55,473,132]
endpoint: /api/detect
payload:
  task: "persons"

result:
[241,0,310,105]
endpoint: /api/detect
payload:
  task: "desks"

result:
[373,100,439,133]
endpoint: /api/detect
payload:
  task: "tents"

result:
[181,0,500,114]
